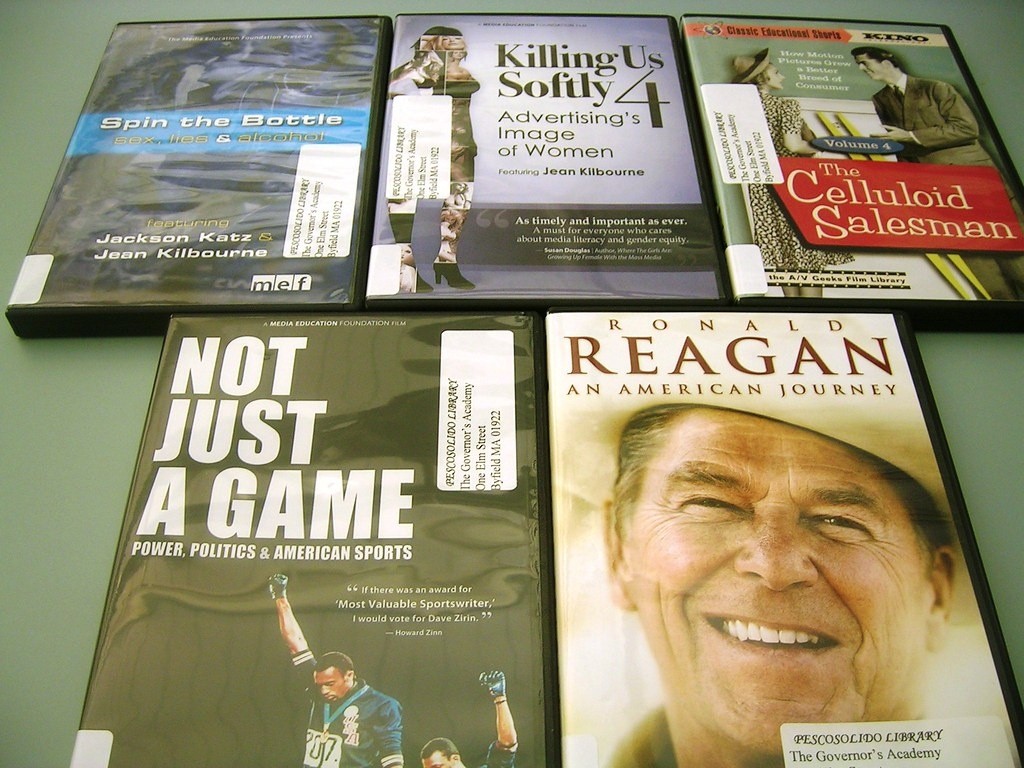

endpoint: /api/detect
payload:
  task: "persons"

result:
[850,46,1024,301]
[594,399,962,768]
[268,573,405,768]
[731,48,855,298]
[387,25,480,293]
[419,671,518,768]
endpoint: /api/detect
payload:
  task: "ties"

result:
[894,85,904,106]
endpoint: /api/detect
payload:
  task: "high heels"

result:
[415,270,432,291]
[433,261,476,291]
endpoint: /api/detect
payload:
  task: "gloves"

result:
[478,671,506,704]
[268,574,289,600]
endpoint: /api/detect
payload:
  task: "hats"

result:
[546,314,975,630]
[732,48,770,84]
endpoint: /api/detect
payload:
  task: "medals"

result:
[320,732,329,743]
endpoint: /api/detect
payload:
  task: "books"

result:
[365,12,727,311]
[546,305,1024,768]
[68,308,551,768]
[680,14,1024,333]
[4,13,392,339]
